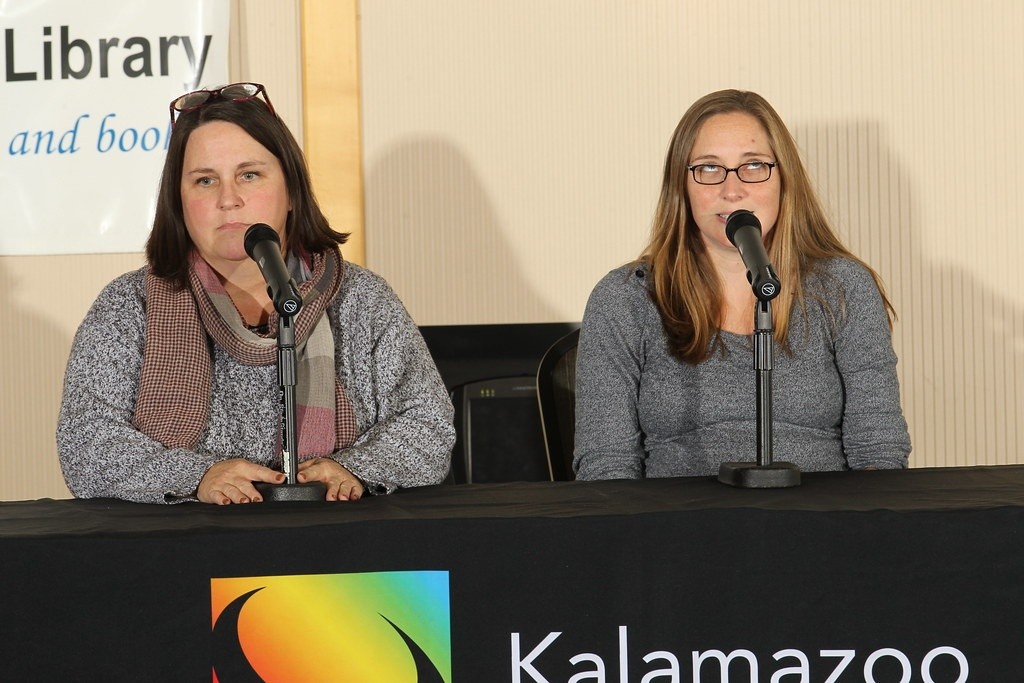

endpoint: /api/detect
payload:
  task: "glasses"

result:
[169,82,277,133]
[687,161,778,185]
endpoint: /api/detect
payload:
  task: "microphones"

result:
[725,209,781,301]
[243,223,303,317]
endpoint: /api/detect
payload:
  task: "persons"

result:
[55,82,457,505]
[572,90,912,481]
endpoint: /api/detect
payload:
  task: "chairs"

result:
[537,327,580,481]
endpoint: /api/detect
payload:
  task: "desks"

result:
[1,463,1024,683]
